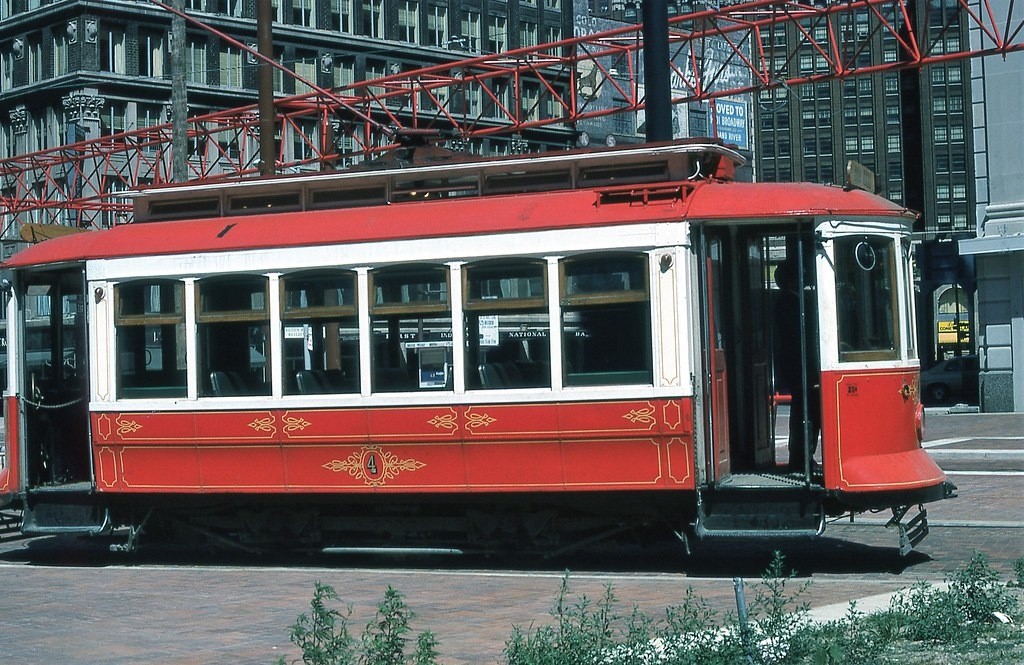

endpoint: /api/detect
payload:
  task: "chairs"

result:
[211,362,584,397]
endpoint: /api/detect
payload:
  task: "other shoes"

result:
[789,459,823,474]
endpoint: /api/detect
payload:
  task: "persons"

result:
[774,260,820,470]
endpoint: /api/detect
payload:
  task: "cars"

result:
[919,355,980,401]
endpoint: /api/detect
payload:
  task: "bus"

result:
[0,135,959,556]
